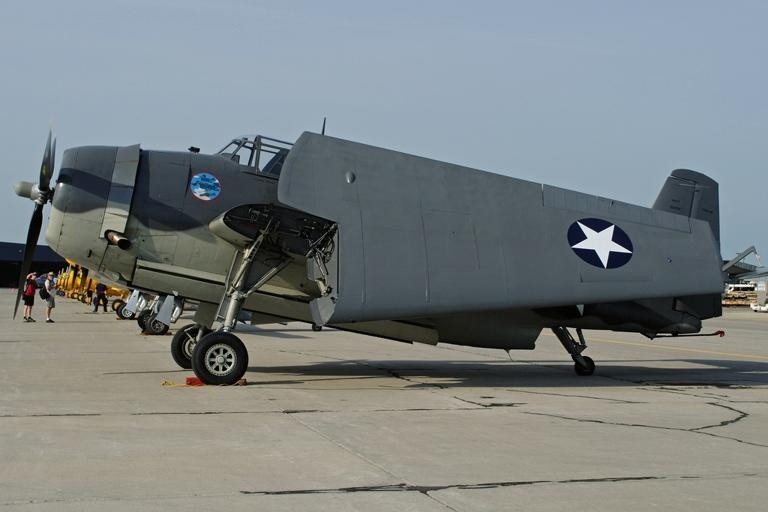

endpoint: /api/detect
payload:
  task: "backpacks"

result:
[23,278,36,297]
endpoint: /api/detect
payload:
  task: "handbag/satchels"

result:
[40,288,50,299]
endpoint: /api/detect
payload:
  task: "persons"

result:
[93,283,109,312]
[44,271,57,323]
[22,272,38,322]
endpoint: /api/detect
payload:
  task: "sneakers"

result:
[23,317,35,322]
[46,319,54,323]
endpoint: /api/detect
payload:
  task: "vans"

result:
[724,284,757,301]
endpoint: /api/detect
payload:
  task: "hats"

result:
[48,272,54,276]
